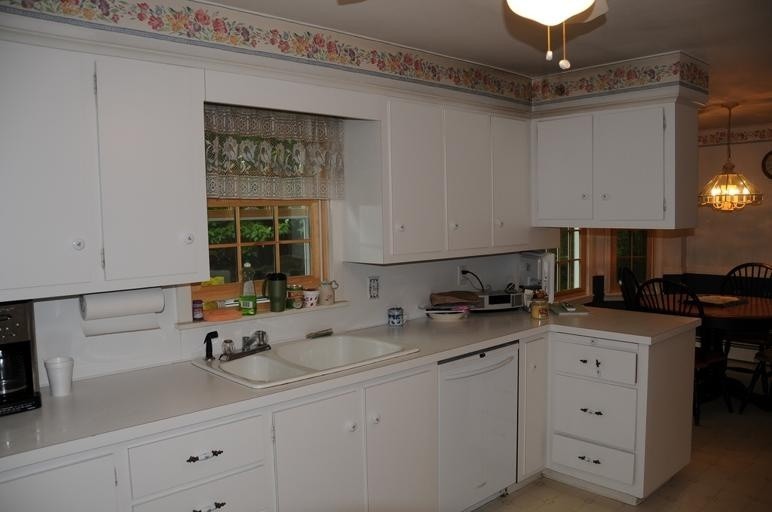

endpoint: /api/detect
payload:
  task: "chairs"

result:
[617,262,772,425]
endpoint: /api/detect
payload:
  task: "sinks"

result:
[190,355,314,389]
[277,334,421,371]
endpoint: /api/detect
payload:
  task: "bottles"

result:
[239,262,256,315]
[318,280,334,307]
[388,307,403,327]
[242,337,250,351]
[192,300,204,321]
[531,299,550,321]
[254,331,266,345]
[223,339,234,354]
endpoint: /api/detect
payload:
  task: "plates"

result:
[424,311,464,313]
[429,313,464,319]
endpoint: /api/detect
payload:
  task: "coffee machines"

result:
[0,301,42,417]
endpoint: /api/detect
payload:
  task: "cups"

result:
[263,273,320,312]
[45,356,74,397]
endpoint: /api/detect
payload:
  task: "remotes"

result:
[561,301,576,312]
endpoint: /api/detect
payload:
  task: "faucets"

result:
[242,330,265,354]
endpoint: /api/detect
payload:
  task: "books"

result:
[550,303,590,316]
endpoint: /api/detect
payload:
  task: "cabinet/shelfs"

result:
[0,454,117,512]
[0,39,211,303]
[547,329,696,506]
[128,415,278,512]
[273,365,438,512]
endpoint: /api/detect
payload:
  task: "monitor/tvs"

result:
[520,251,555,304]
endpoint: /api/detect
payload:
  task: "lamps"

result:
[698,102,763,213]
[507,0,608,70]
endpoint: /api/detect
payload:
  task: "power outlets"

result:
[458,266,467,286]
